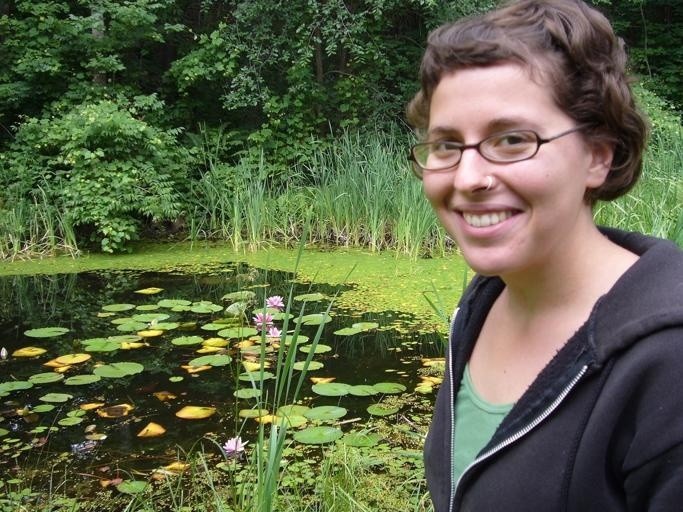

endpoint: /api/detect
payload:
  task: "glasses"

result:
[405,123,595,172]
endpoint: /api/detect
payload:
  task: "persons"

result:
[399,0,682,512]
